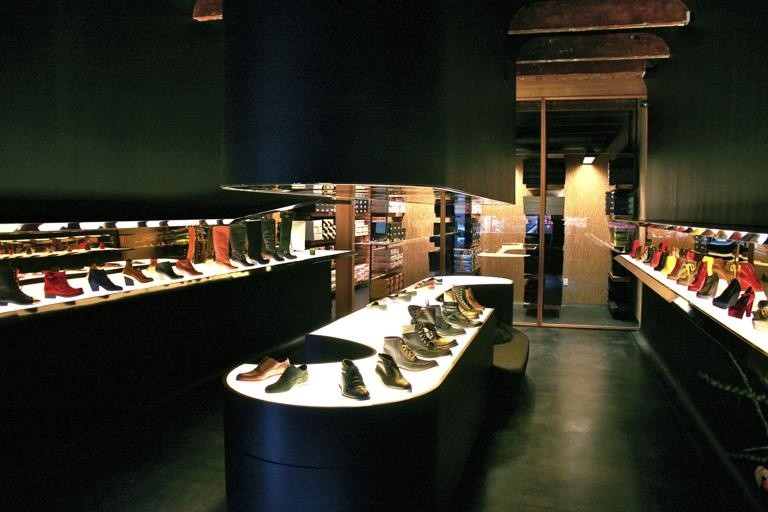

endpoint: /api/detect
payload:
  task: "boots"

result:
[230,224,255,267]
[211,225,239,271]
[277,217,297,260]
[0,264,33,306]
[247,220,270,265]
[262,219,285,262]
[44,271,84,298]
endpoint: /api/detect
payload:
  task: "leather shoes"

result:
[375,353,413,394]
[265,364,309,394]
[237,354,291,382]
[382,288,485,372]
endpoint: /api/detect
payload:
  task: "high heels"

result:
[628,238,767,330]
[123,265,154,286]
[155,262,184,280]
[176,259,203,276]
[88,269,123,292]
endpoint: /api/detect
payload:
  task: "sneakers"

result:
[338,359,370,400]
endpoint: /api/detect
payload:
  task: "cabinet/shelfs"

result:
[294,184,481,321]
[523,153,639,320]
